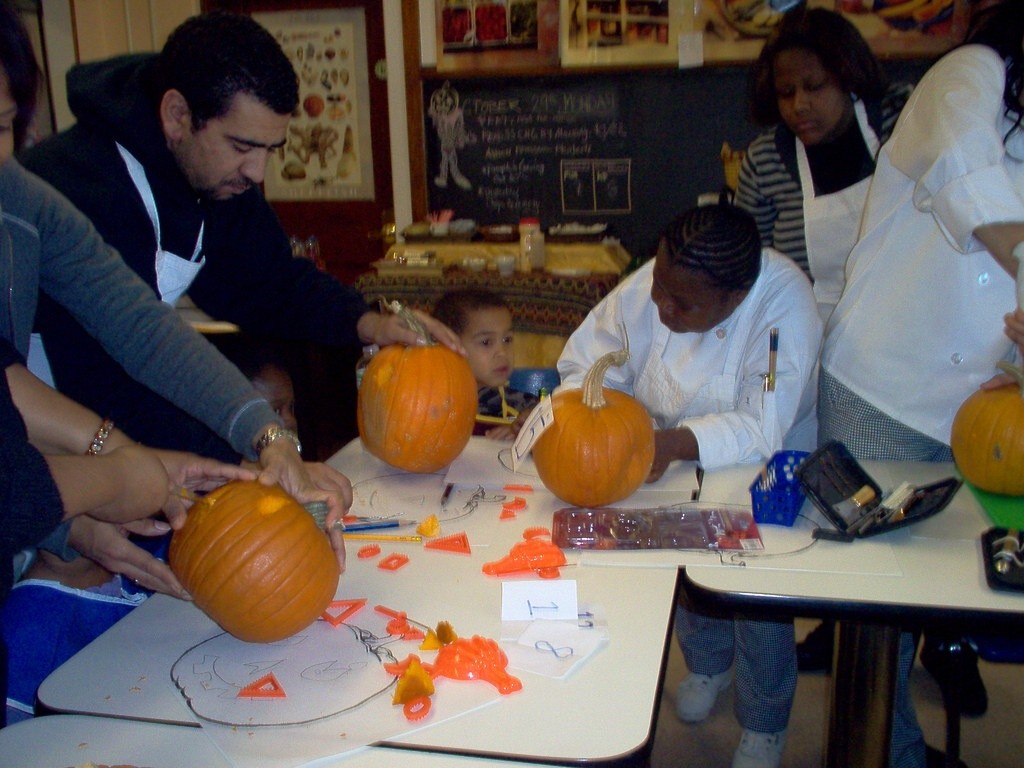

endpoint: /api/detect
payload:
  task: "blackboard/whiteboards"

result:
[421,54,938,244]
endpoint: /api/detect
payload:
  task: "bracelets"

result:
[85,419,113,455]
[256,426,302,456]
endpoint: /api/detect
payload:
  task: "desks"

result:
[0,436,1024,768]
[176,298,241,336]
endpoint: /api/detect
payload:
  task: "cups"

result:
[497,255,515,276]
[430,222,449,235]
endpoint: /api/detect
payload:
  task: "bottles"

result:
[519,219,540,274]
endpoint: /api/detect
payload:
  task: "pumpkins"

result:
[358,300,479,475]
[950,362,1024,495]
[531,348,655,507]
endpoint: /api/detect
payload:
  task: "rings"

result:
[133,578,140,584]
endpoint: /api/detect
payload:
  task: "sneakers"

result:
[730,729,790,768]
[676,667,731,723]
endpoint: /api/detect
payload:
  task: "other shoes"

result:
[919,640,990,717]
[795,640,838,674]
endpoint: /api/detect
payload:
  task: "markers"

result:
[397,250,438,266]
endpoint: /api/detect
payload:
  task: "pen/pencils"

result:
[764,373,770,391]
[342,532,421,541]
[440,483,453,505]
[343,519,417,531]
[178,488,208,504]
[769,327,778,390]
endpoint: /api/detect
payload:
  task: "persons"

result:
[0,0,354,722]
[512,203,820,768]
[430,290,540,438]
[20,11,469,460]
[734,0,1024,768]
